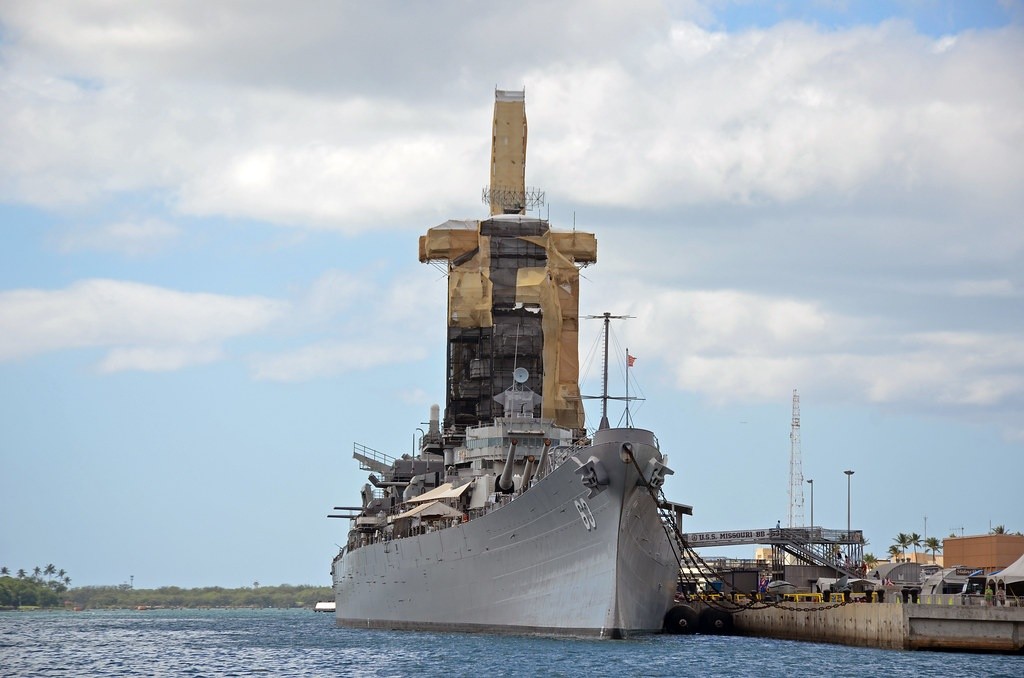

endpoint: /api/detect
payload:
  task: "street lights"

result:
[807,478,814,554]
[844,470,855,562]
[923,515,928,553]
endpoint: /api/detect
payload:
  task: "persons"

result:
[997,585,1006,606]
[984,584,993,606]
[347,530,392,547]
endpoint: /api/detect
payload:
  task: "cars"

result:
[313,600,336,612]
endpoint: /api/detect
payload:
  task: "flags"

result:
[628,355,637,366]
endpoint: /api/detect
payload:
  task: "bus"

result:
[734,594,762,604]
[783,593,824,603]
[690,594,723,603]
[829,593,879,603]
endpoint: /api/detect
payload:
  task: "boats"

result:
[330,82,693,644]
